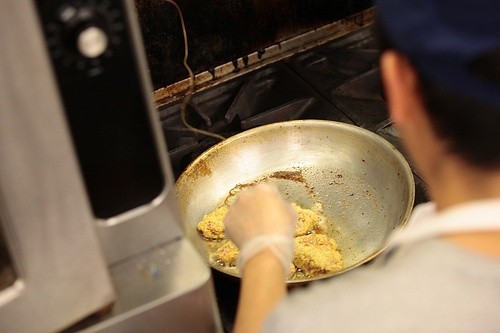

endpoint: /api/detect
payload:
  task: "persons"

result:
[228,0,500,333]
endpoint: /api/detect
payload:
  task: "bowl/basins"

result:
[173,121,415,287]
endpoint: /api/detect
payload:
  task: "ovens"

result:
[135,0,434,215]
